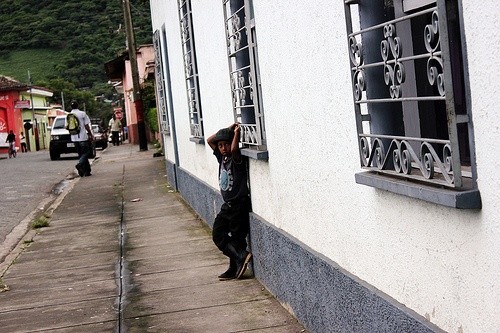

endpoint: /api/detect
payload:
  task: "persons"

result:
[19,132,27,152]
[6,130,16,154]
[67,101,94,176]
[207,123,252,280]
[109,114,121,146]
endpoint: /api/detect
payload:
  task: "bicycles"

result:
[4,140,16,158]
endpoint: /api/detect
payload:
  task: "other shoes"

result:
[75,162,91,177]
[117,144,119,146]
[113,144,115,146]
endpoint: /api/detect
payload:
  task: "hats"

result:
[214,128,234,141]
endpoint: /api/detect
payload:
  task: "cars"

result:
[90,125,108,147]
[49,114,96,158]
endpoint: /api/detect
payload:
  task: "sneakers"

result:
[218,268,235,280]
[234,251,253,280]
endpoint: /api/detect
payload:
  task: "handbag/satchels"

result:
[66,112,81,138]
